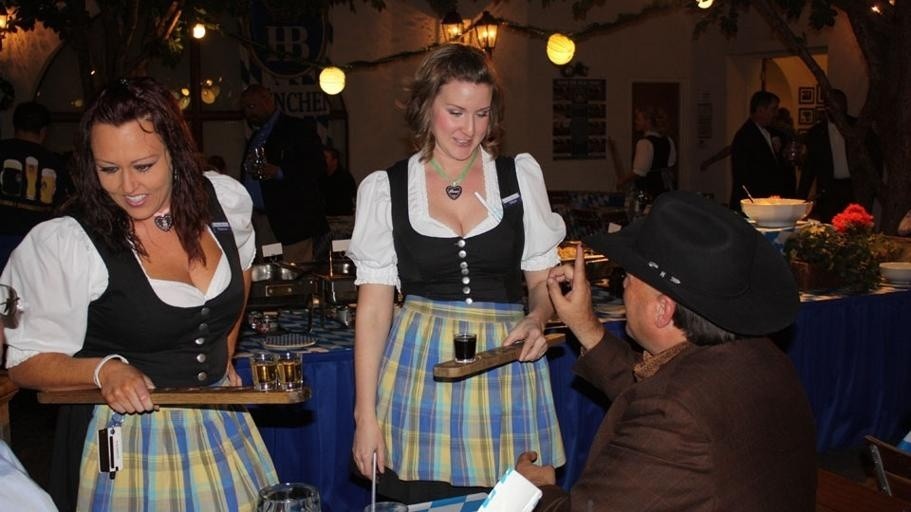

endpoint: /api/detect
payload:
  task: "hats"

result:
[583,190,799,335]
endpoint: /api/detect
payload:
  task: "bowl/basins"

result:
[740,196,809,228]
[878,261,911,281]
[254,316,279,334]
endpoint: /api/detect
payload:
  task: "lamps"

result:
[439,3,499,58]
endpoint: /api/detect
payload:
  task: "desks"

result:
[1,186,911,510]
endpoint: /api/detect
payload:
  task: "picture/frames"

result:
[795,81,830,169]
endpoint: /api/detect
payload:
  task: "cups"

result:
[258,480,321,511]
[365,500,412,511]
[453,329,478,364]
[0,156,57,204]
[251,350,306,394]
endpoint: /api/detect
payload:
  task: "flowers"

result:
[789,202,884,295]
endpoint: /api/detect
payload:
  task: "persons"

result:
[728,88,894,225]
[238,83,328,244]
[344,43,568,504]
[321,147,357,216]
[614,107,679,203]
[514,189,819,512]
[0,99,71,273]
[0,73,281,512]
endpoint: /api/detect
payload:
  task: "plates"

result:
[594,305,628,318]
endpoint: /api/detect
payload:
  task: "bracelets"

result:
[91,353,129,388]
